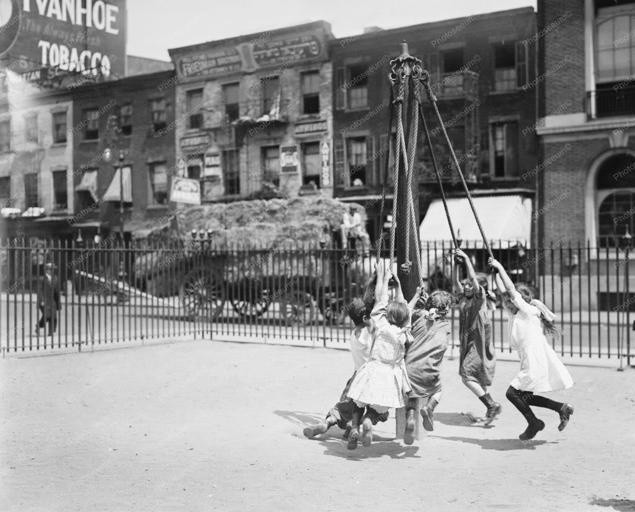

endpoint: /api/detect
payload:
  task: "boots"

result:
[478,393,501,425]
[403,396,439,445]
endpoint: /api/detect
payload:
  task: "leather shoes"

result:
[519,420,544,440]
[558,401,574,432]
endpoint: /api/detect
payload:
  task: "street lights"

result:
[116,151,131,306]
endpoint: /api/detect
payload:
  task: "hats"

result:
[43,262,58,270]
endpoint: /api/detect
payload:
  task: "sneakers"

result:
[346,418,371,450]
[303,424,330,437]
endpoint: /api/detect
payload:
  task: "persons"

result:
[33,261,63,337]
[455,249,502,426]
[340,203,370,258]
[303,257,456,451]
[488,258,573,441]
